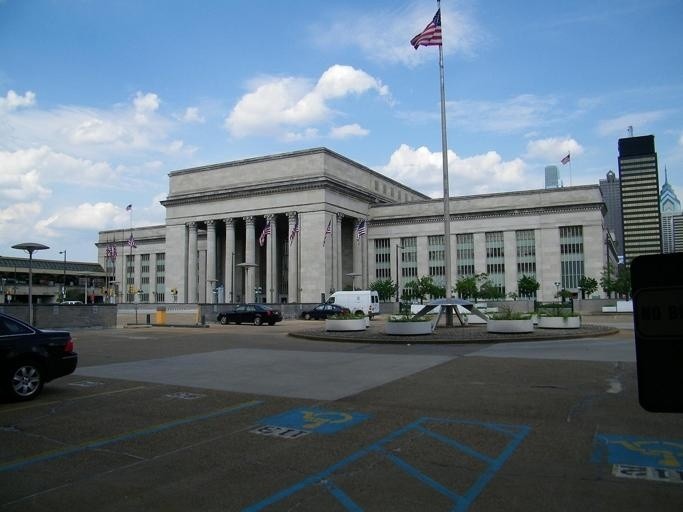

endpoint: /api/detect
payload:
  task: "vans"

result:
[323,290,381,319]
[59,300,83,306]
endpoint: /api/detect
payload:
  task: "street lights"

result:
[553,280,560,303]
[10,243,49,326]
[344,272,362,291]
[77,272,94,304]
[602,233,614,298]
[395,244,406,302]
[206,249,263,305]
[58,249,67,301]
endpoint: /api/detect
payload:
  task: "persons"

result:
[368,307,372,320]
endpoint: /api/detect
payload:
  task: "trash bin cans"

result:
[156,306,166,324]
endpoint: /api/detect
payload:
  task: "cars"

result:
[301,303,349,320]
[215,303,282,326]
[0,311,77,400]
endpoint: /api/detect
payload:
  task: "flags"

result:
[127,235,136,248]
[126,204,131,211]
[355,219,366,239]
[289,223,299,246]
[258,221,271,247]
[322,219,331,247]
[561,154,570,165]
[109,245,118,262]
[409,8,443,51]
